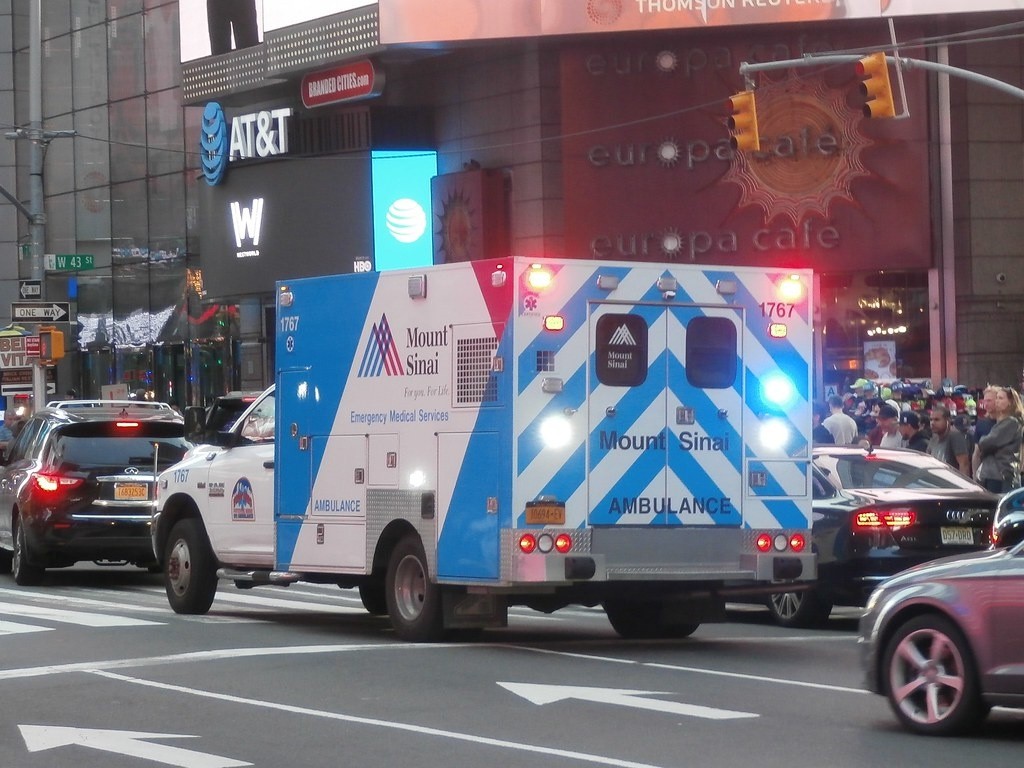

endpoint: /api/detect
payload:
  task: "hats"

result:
[966,398,977,416]
[843,392,852,402]
[849,378,868,389]
[893,411,919,424]
[891,380,904,391]
[882,387,891,399]
[874,405,898,417]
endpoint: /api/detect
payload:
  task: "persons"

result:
[169,400,182,417]
[63,388,83,408]
[129,388,155,408]
[0,408,20,462]
[812,378,1024,494]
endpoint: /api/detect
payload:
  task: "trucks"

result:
[151,256,818,642]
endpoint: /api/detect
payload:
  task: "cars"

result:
[723,442,1001,631]
[855,489,1024,736]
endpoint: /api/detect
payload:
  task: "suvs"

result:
[203,390,265,444]
[0,400,198,587]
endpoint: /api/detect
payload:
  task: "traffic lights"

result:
[725,89,762,153]
[38,330,64,360]
[855,52,895,122]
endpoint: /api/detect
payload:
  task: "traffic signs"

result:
[18,280,42,299]
[11,301,72,323]
